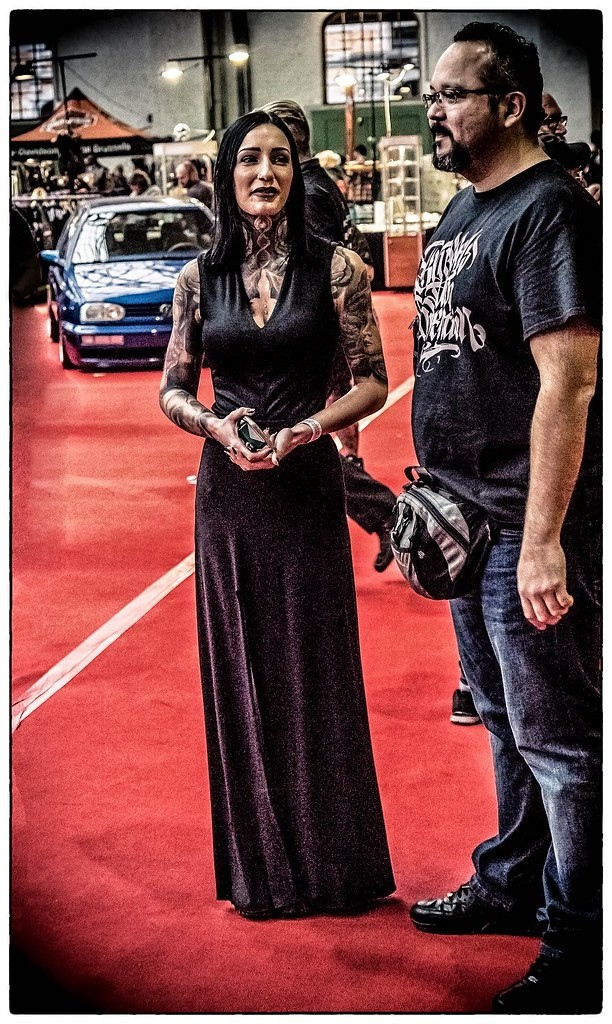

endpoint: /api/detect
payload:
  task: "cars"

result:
[42,196,221,373]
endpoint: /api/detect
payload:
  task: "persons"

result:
[250,98,402,571]
[13,142,390,291]
[158,112,399,920]
[410,21,604,1017]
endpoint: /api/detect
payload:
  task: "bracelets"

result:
[296,417,323,445]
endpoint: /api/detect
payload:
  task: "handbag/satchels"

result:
[387,466,492,599]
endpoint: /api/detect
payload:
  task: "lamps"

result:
[161,54,225,80]
[226,43,251,63]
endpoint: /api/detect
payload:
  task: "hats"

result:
[542,94,562,120]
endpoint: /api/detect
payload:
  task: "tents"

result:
[9,85,146,196]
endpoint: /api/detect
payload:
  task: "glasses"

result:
[544,116,567,129]
[422,88,499,109]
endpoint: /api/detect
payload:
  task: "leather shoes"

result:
[375,508,394,573]
[492,957,578,1012]
[410,881,525,934]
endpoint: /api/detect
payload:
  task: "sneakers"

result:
[450,689,479,724]
